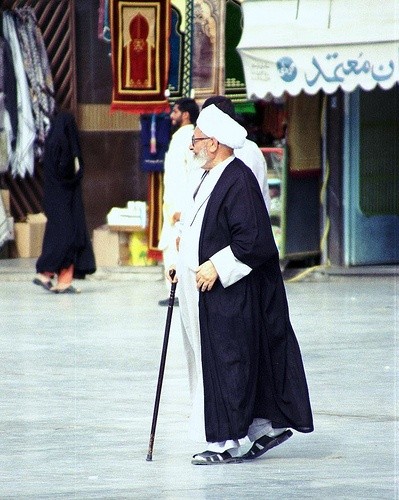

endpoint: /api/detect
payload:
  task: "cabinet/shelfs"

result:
[262,147,320,271]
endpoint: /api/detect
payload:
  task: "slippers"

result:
[243,429,294,460]
[192,449,244,465]
[33,278,54,291]
[50,285,81,294]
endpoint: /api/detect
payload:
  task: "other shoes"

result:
[158,297,180,306]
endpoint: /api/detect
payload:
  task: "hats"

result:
[196,104,248,149]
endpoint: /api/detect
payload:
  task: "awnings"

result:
[236,0,398,100]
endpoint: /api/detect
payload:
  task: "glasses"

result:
[192,135,219,148]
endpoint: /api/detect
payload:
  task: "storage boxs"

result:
[92,225,130,267]
[10,213,47,258]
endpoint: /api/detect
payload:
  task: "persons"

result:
[166,104,314,466]
[32,111,96,295]
[159,96,267,306]
